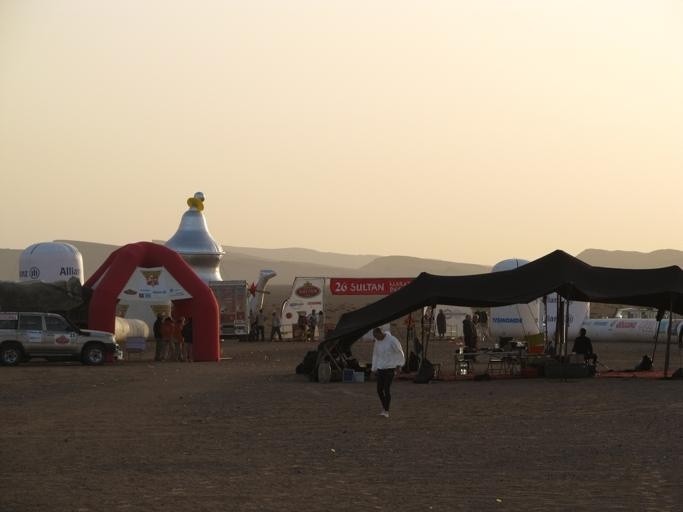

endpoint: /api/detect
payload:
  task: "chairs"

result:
[425,333,598,384]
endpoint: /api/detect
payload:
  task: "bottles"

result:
[458,342,463,355]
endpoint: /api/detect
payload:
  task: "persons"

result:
[153,313,192,363]
[317,311,324,336]
[254,309,265,341]
[271,312,282,340]
[437,309,446,338]
[463,311,489,350]
[572,328,597,372]
[308,309,316,341]
[371,328,406,418]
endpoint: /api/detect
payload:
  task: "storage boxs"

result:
[354,373,366,383]
[343,368,354,383]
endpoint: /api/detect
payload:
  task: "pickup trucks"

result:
[1,312,125,367]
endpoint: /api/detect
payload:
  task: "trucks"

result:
[207,279,250,345]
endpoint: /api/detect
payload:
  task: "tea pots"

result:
[165,192,276,321]
